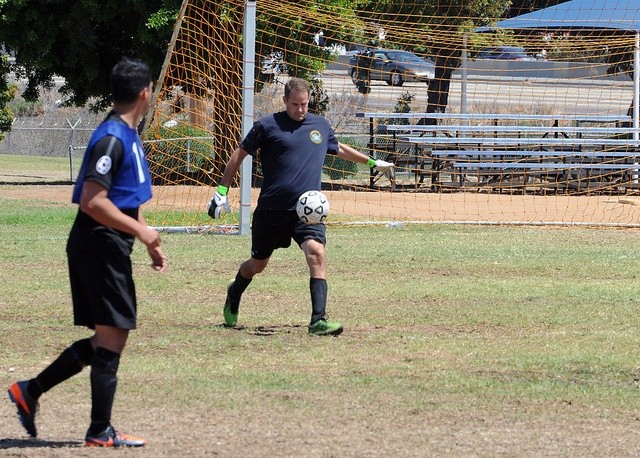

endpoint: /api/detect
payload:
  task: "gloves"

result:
[367,155,395,181]
[206,184,231,219]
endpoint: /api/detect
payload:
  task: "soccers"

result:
[295,190,330,225]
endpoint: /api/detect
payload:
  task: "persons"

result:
[5,56,168,449]
[206,77,397,337]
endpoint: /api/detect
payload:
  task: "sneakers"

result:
[308,317,343,335]
[85,426,148,448]
[223,278,242,326]
[8,379,38,437]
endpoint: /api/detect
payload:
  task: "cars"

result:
[348,48,436,86]
[473,46,536,61]
